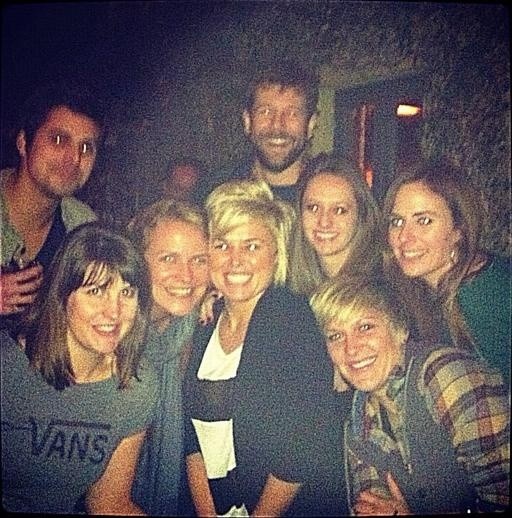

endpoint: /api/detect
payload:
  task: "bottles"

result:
[10,241,31,312]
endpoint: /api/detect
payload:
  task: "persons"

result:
[115,191,223,517]
[304,269,511,516]
[0,221,165,516]
[173,174,353,518]
[369,155,512,393]
[1,85,110,348]
[281,147,391,422]
[186,56,324,212]
